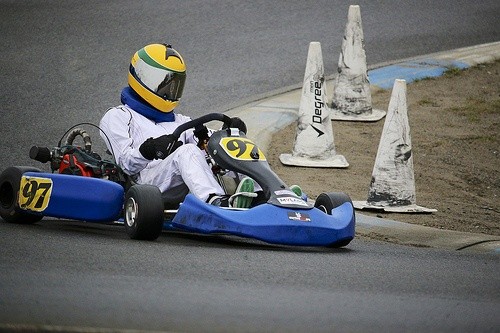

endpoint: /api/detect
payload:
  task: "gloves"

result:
[222,117,247,135]
[139,133,183,160]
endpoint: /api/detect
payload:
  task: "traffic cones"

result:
[352,78,439,214]
[278,41,350,169]
[329,4,386,121]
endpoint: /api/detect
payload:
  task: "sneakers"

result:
[290,185,302,197]
[210,178,255,208]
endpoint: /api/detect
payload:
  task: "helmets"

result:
[128,42,187,112]
